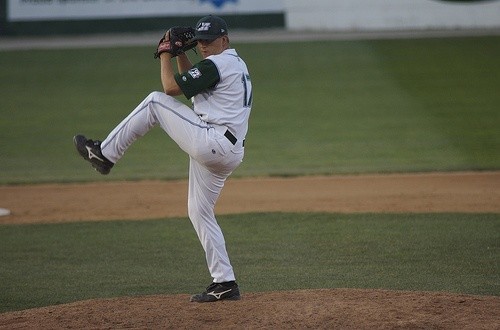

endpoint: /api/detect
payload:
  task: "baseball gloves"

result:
[153,25,199,58]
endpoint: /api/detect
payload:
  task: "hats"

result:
[191,14,228,41]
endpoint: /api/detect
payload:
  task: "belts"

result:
[224,130,245,148]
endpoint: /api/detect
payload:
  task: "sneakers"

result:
[190,283,240,302]
[73,134,114,175]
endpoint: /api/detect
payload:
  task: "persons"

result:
[73,13,253,303]
